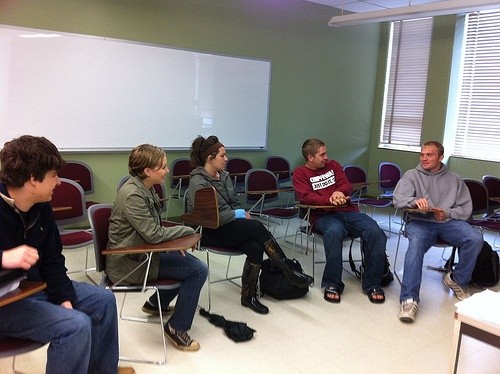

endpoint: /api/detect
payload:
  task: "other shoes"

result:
[118,367,135,374]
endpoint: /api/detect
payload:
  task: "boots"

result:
[263,236,314,288]
[241,260,269,313]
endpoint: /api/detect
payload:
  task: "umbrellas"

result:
[197,305,256,343]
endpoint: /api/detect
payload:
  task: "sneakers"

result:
[444,271,471,300]
[163,320,200,350]
[399,297,417,322]
[143,301,176,315]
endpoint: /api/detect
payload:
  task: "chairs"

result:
[0,156,500,374]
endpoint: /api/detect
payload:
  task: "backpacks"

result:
[472,241,500,286]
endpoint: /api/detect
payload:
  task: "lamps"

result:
[328,0,500,28]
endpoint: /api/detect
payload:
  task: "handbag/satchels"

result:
[259,255,309,300]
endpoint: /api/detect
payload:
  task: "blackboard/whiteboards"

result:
[0,24,272,154]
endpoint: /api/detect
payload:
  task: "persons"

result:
[393,141,483,321]
[188,135,307,314]
[106,143,208,351]
[0,135,135,374]
[293,138,387,303]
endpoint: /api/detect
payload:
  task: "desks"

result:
[449,311,500,374]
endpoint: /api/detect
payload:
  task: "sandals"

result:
[324,285,340,302]
[367,288,385,303]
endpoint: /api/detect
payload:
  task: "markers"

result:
[260,147,264,148]
